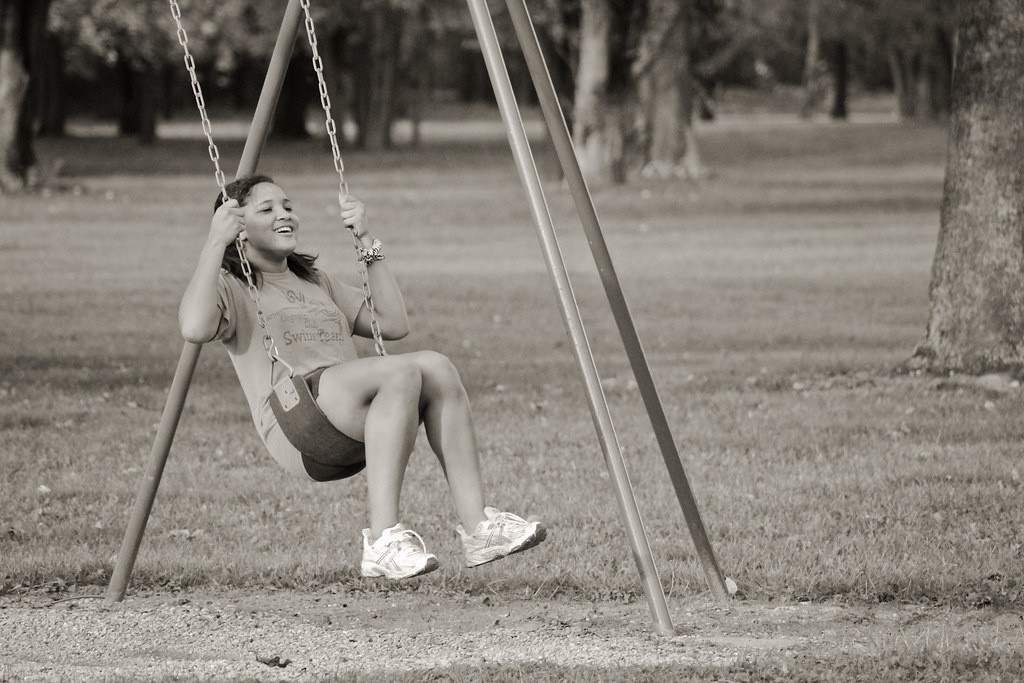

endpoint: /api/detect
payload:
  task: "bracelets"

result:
[360,237,385,266]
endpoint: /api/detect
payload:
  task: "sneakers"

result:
[359,523,438,580]
[456,506,549,570]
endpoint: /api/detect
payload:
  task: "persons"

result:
[177,172,547,579]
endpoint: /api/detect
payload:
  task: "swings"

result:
[170,0,388,463]
